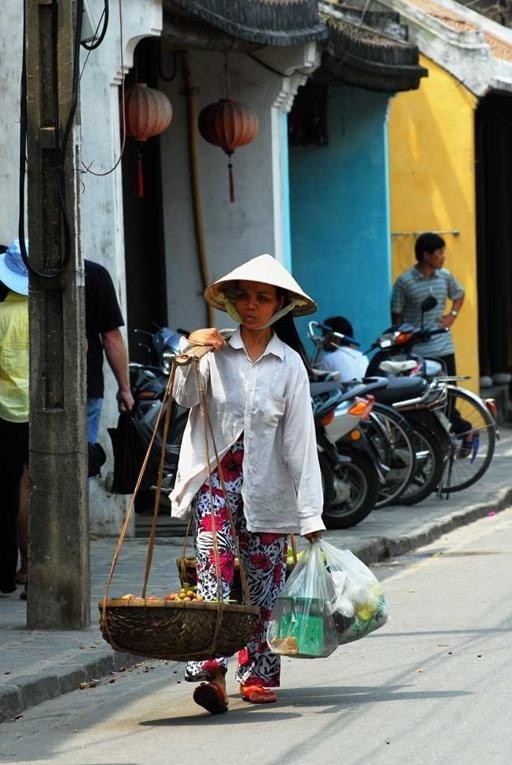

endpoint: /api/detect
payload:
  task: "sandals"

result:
[240,677,277,703]
[193,681,227,714]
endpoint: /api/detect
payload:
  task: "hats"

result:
[0,238,29,295]
[203,254,318,317]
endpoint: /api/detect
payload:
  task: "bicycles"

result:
[307,320,500,499]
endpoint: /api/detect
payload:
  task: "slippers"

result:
[458,430,477,457]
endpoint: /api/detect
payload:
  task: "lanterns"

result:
[120,82,172,200]
[198,97,258,203]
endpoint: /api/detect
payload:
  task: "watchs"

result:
[450,310,457,317]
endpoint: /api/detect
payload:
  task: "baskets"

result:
[98,600,261,661]
[176,557,296,603]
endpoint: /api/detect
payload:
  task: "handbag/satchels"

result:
[107,403,167,495]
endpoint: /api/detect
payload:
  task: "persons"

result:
[172,252,326,714]
[0,244,33,592]
[19,256,134,603]
[390,231,479,460]
